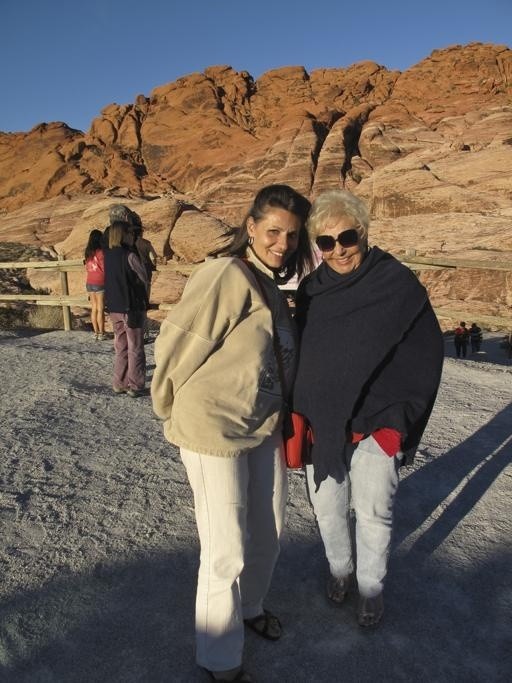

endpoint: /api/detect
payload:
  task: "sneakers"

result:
[128,388,149,398]
[113,385,130,392]
[92,332,112,340]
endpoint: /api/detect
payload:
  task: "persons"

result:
[84,202,158,398]
[281,187,446,634]
[468,322,482,353]
[453,320,469,359]
[148,181,317,683]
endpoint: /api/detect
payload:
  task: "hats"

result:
[109,206,131,224]
[132,212,145,229]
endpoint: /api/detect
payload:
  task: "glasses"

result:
[316,228,360,251]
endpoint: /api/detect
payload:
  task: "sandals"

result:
[204,667,252,683]
[247,608,281,641]
[358,592,384,625]
[325,574,351,603]
